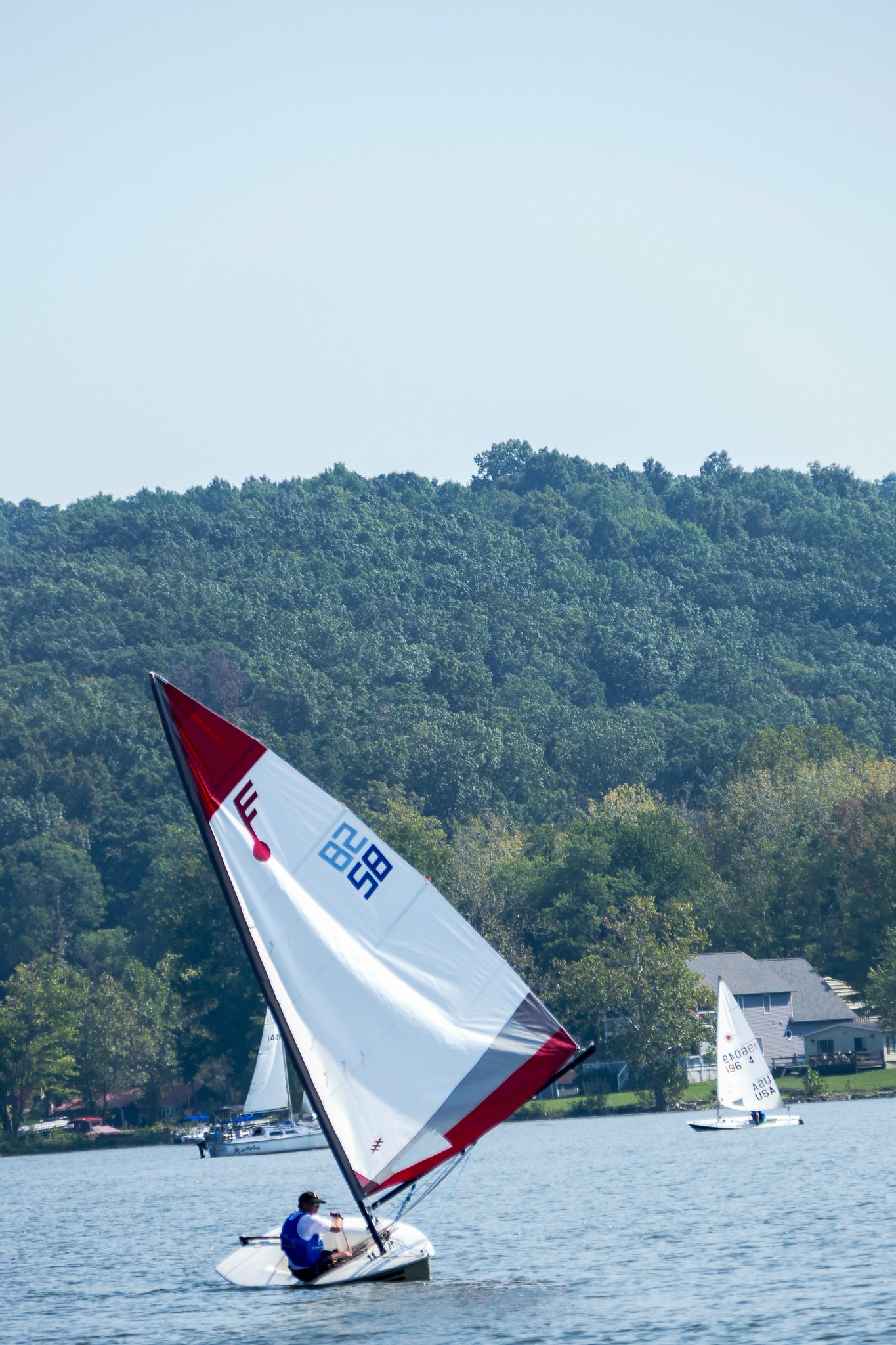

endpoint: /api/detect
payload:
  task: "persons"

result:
[750,1111,765,1125]
[280,1191,351,1282]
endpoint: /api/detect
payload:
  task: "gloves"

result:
[756,1122,759,1126]
[330,1212,344,1220]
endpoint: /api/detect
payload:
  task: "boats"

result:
[171,1105,269,1145]
[18,1116,123,1139]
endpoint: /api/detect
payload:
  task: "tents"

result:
[177,1105,255,1141]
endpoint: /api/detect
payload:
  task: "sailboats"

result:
[197,1006,329,1158]
[297,1091,319,1128]
[685,975,805,1132]
[149,670,597,1289]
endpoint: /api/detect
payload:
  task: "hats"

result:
[299,1191,326,1204]
